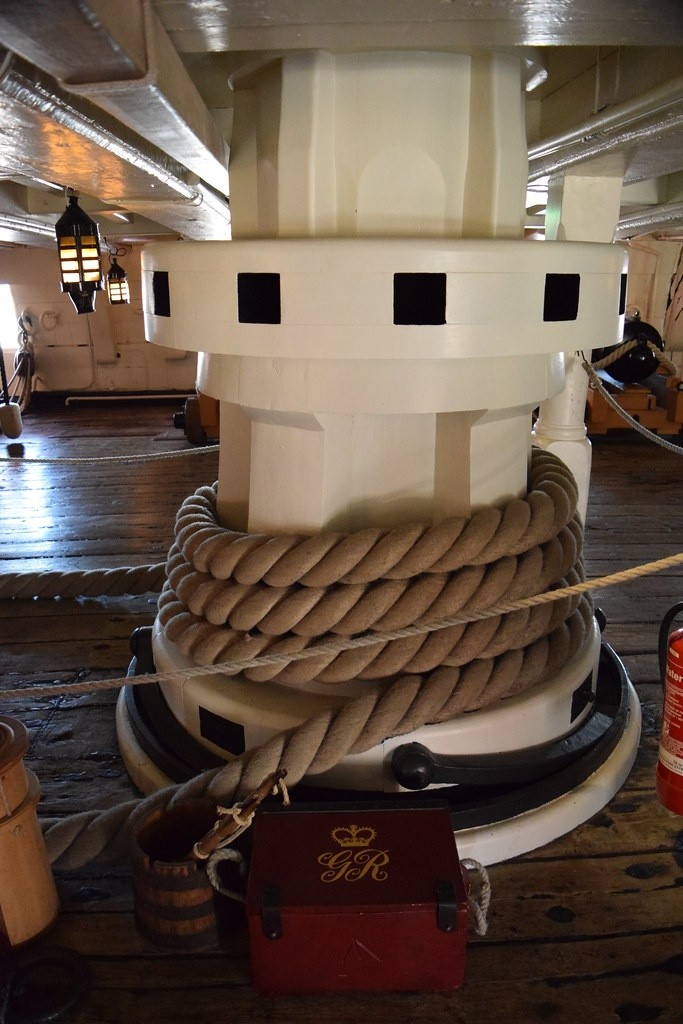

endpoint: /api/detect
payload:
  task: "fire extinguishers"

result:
[654,601,683,816]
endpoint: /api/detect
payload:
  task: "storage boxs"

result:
[243,805,470,996]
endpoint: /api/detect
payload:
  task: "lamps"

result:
[105,247,131,305]
[50,186,106,315]
